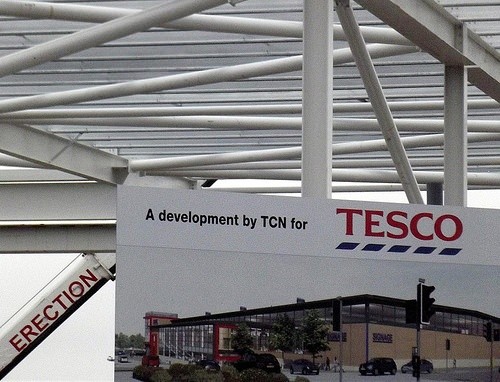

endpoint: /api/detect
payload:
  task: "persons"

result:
[453,357,457,368]
[327,357,331,371]
[334,357,338,372]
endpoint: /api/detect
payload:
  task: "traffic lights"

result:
[420,283,436,326]
[482,322,492,341]
[329,298,340,332]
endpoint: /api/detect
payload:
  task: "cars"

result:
[118,355,128,363]
[288,359,320,375]
[195,360,220,374]
[401,358,433,373]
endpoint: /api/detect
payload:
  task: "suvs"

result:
[359,357,398,376]
[230,353,281,375]
